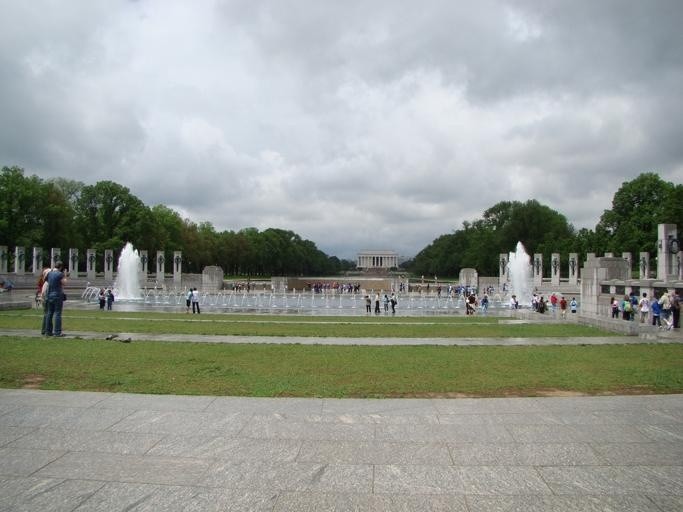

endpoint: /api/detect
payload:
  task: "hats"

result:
[42,268,51,279]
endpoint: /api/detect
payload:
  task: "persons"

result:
[374,293,381,313]
[389,293,398,313]
[0,278,14,292]
[97,288,114,310]
[231,280,250,293]
[35,261,69,337]
[570,297,578,314]
[398,282,406,293]
[510,295,519,309]
[185,288,193,314]
[549,292,558,313]
[610,290,683,332]
[532,292,549,314]
[559,297,567,319]
[305,279,360,294]
[364,295,371,315]
[416,282,494,315]
[382,295,390,313]
[192,288,200,314]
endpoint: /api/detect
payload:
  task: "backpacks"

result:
[624,302,632,312]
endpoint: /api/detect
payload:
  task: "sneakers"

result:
[665,322,674,330]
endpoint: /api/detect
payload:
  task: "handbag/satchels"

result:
[34,293,47,312]
[671,303,680,312]
